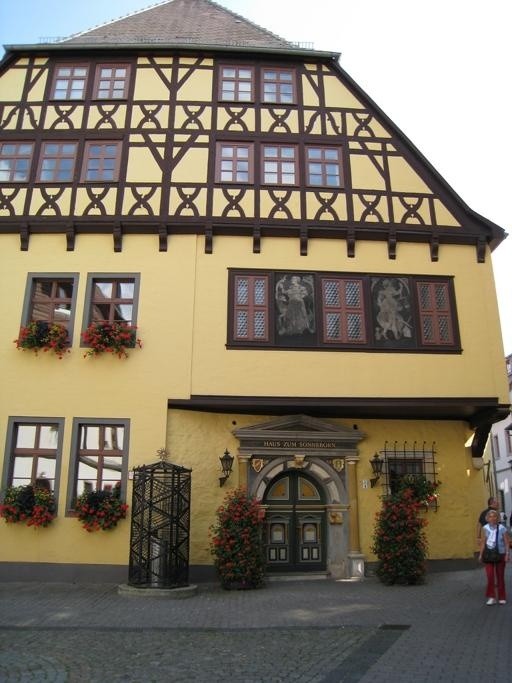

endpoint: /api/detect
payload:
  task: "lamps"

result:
[217,448,235,487]
[369,451,384,488]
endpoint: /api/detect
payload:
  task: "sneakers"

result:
[499,599,506,604]
[486,598,496,605]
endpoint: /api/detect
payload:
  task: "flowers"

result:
[208,483,267,590]
[1,484,58,529]
[81,320,142,360]
[75,488,129,534]
[13,318,71,360]
[369,472,442,586]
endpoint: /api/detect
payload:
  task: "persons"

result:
[275,274,314,338]
[478,509,510,606]
[499,510,506,527]
[376,280,403,341]
[477,497,500,545]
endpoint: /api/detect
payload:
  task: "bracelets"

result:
[505,552,510,554]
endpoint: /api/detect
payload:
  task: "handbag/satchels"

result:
[482,545,498,564]
